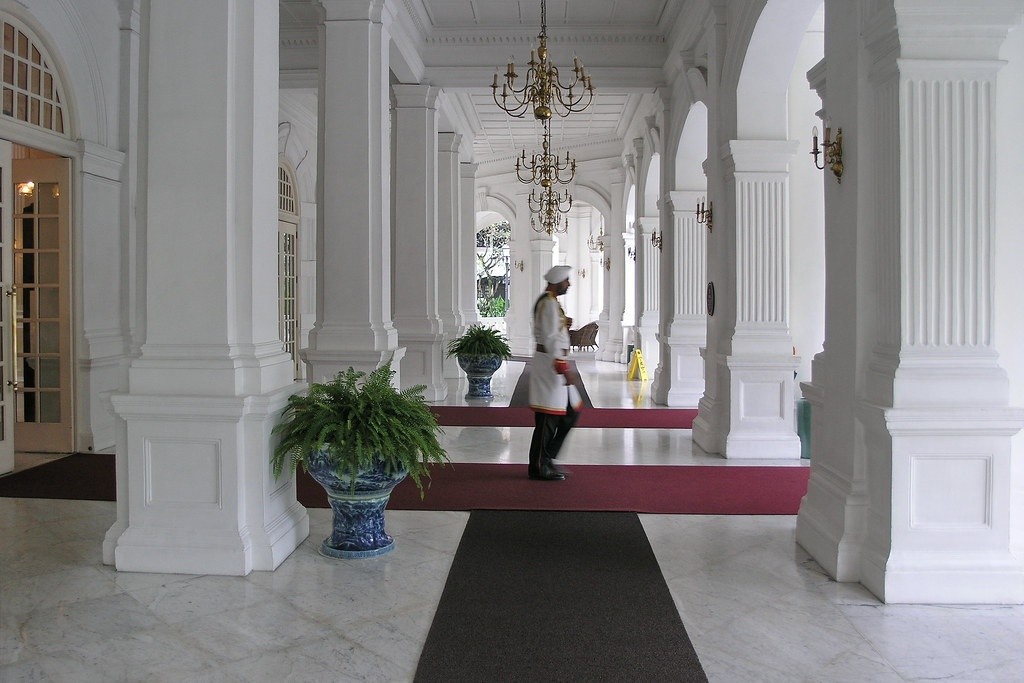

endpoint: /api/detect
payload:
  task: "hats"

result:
[544,266,571,284]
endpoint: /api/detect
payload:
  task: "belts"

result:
[537,343,570,357]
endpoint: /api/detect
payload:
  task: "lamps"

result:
[531,209,569,235]
[696,196,714,231]
[528,183,574,217]
[492,0,597,126]
[651,230,664,252]
[514,118,579,189]
[601,258,610,271]
[627,247,636,261]
[587,228,609,251]
[514,261,524,272]
[577,268,586,278]
[813,116,845,183]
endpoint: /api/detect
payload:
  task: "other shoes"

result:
[529,460,567,479]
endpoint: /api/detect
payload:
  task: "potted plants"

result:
[446,324,512,400]
[269,363,453,559]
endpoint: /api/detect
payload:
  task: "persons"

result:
[528,266,583,480]
